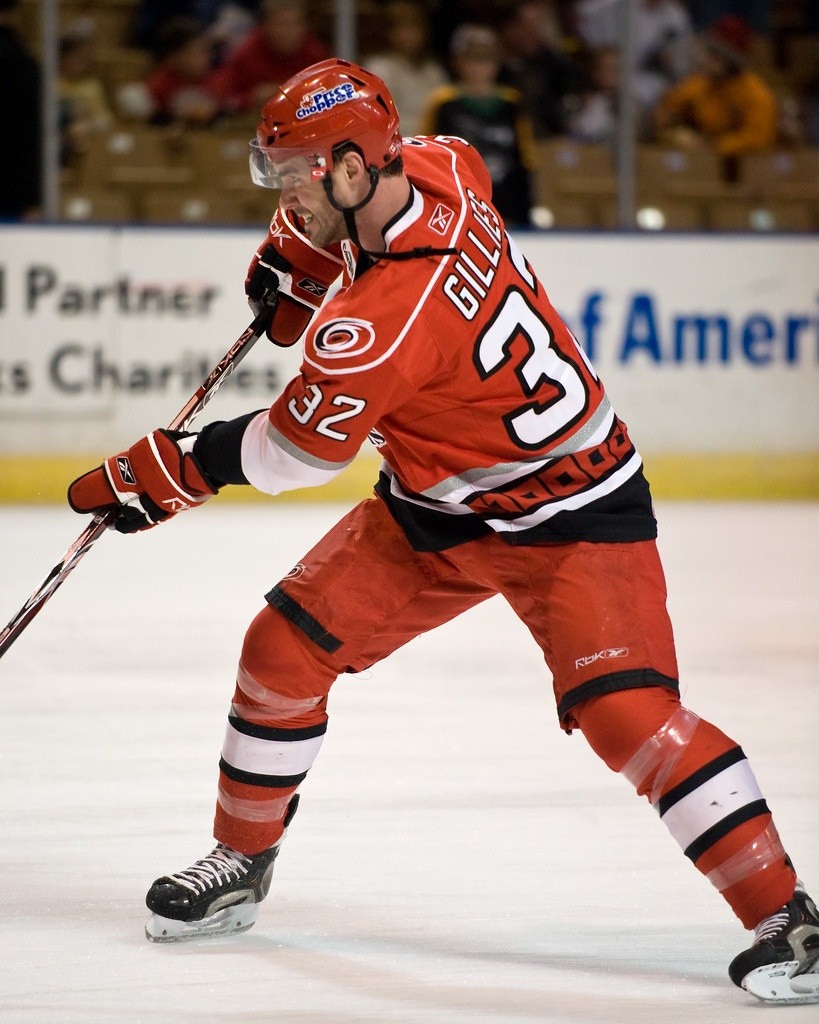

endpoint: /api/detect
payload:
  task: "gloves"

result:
[68,428,219,535]
[245,208,345,347]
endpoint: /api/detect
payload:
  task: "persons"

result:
[120,0,819,229]
[65,57,819,1005]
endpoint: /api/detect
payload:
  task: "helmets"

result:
[257,57,403,175]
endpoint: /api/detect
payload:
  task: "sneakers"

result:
[145,794,301,941]
[728,888,818,1003]
